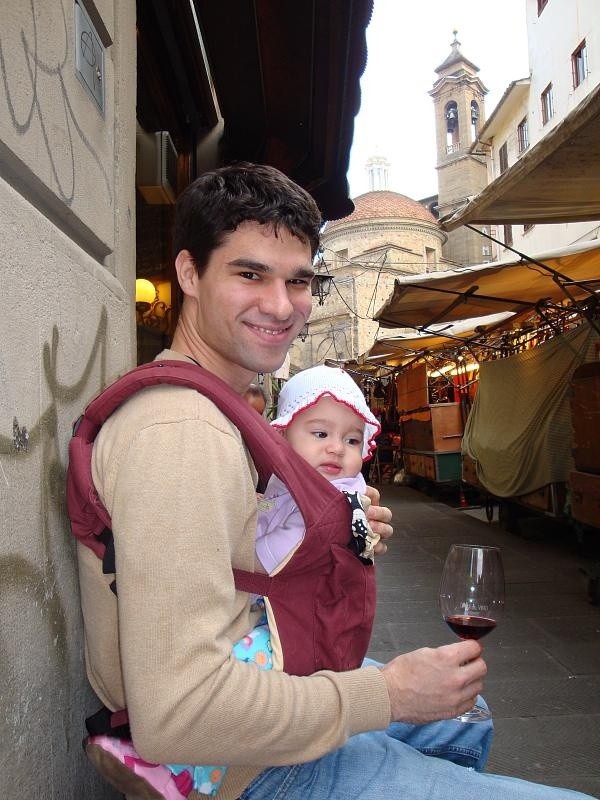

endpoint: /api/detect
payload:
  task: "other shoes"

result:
[85,735,194,800]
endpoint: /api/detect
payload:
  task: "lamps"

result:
[136,278,170,340]
[296,321,311,342]
[311,248,334,306]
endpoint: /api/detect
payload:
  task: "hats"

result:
[269,365,381,463]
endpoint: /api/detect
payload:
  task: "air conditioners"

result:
[137,131,179,205]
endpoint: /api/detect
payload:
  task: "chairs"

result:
[370,444,399,487]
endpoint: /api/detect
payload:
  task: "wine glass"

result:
[438,544,505,723]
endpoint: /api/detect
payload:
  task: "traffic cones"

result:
[455,484,469,508]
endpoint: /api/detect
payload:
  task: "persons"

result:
[64,162,600,800]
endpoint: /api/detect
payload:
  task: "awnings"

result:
[326,87,600,382]
